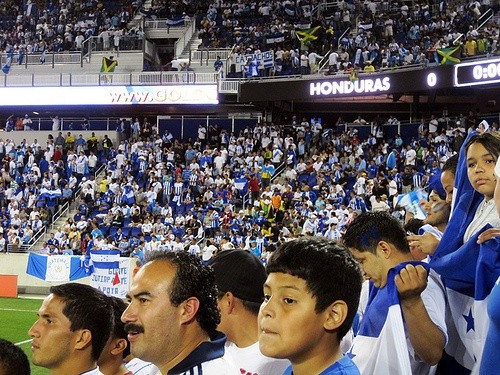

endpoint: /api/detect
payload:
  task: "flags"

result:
[1,53,13,74]
[436,46,462,64]
[165,17,185,27]
[295,26,323,43]
[100,56,117,82]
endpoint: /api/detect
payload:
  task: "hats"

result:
[330,223,338,227]
[311,215,317,219]
[208,249,267,304]
[380,194,388,199]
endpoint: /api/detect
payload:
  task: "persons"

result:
[343,211,449,375]
[121,251,239,375]
[164,57,184,82]
[0,0,236,64]
[200,249,291,375]
[215,55,222,79]
[96,296,136,375]
[0,110,406,264]
[256,235,362,375]
[227,0,500,81]
[403,113,500,375]
[28,283,115,375]
[103,56,118,83]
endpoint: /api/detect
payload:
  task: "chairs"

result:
[0,0,500,256]
